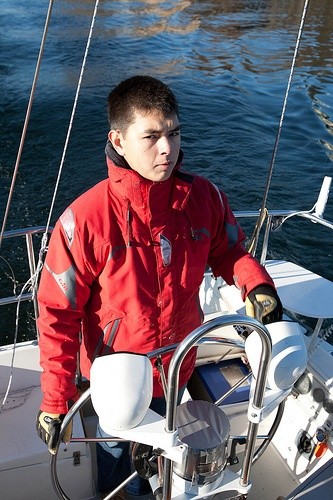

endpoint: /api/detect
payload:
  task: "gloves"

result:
[35,410,73,454]
[246,283,283,325]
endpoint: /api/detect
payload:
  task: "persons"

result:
[35,75,284,500]
